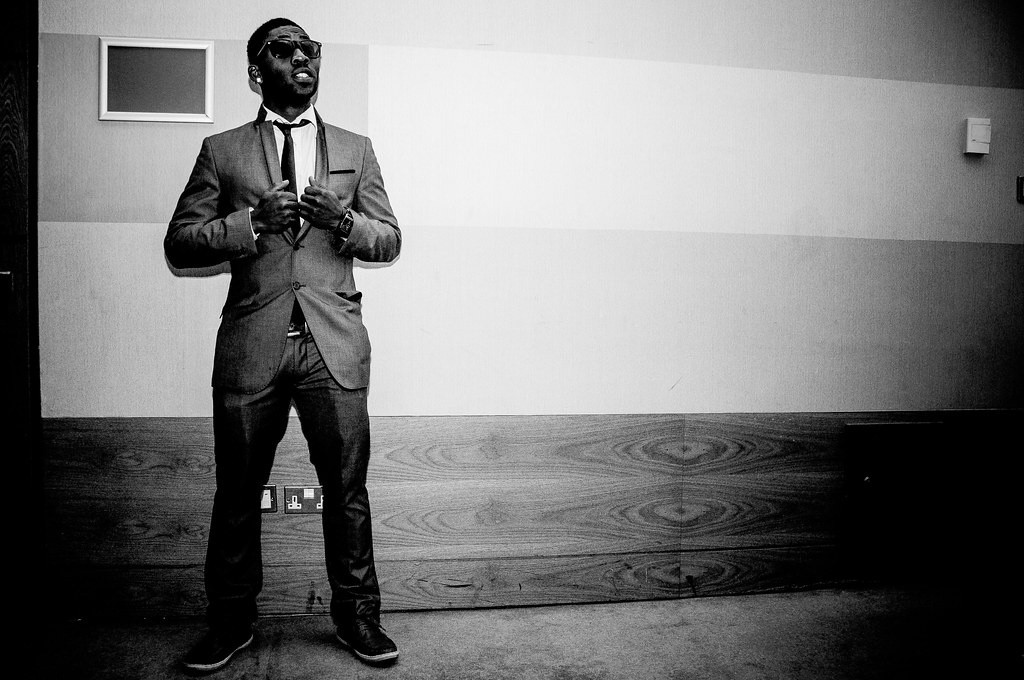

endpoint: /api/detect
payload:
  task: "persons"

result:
[164,18,401,668]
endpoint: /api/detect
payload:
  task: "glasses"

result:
[256,40,322,59]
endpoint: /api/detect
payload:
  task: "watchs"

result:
[338,212,353,235]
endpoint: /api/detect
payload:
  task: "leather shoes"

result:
[183,625,254,672]
[337,615,400,662]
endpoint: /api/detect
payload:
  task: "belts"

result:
[286,320,309,338]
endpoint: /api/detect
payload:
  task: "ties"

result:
[272,119,310,239]
